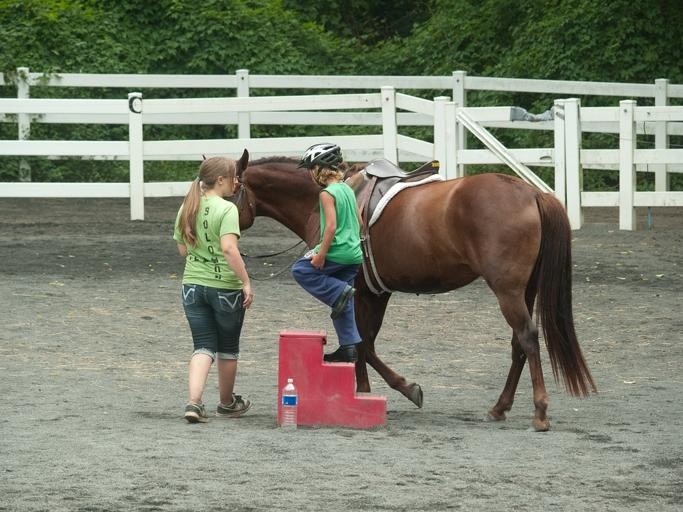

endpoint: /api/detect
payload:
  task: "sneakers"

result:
[216,394,252,417]
[184,402,211,423]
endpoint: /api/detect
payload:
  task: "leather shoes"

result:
[324,345,358,362]
[330,285,358,320]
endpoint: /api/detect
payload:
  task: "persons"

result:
[172,158,255,424]
[291,143,366,363]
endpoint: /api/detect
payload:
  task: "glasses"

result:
[226,175,240,180]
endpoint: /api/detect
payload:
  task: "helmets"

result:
[296,143,343,169]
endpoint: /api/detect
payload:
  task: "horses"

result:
[201,148,599,434]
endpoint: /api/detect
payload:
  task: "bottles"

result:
[279,377,299,430]
